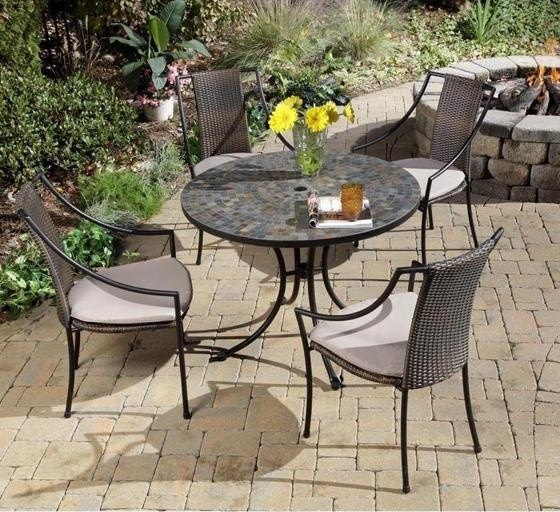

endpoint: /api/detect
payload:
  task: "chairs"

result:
[349,67,499,272]
[169,68,300,268]
[10,168,196,424]
[288,222,503,494]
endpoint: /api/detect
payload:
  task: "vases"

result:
[294,121,327,178]
[143,99,175,123]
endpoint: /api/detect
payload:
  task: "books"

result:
[305,188,374,231]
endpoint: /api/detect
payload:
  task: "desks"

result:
[177,148,427,441]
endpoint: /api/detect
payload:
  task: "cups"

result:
[341,182,364,221]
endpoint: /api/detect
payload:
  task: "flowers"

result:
[132,62,192,108]
[268,95,357,134]
